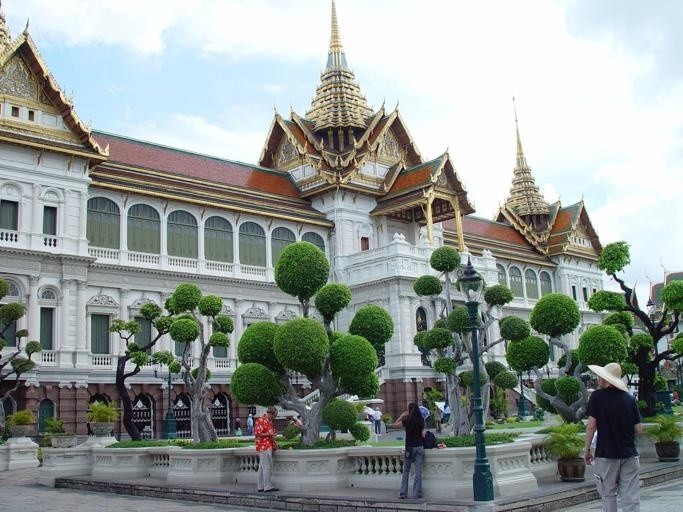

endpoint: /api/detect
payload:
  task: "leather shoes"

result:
[257,487,280,492]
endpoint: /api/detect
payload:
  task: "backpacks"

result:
[423,430,437,449]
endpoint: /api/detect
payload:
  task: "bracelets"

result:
[583,447,591,453]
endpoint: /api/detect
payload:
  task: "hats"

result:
[587,362,630,393]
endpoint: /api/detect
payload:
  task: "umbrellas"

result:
[418,404,430,421]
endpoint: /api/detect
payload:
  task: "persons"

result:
[254,405,278,491]
[234,416,242,436]
[433,407,441,434]
[399,403,424,499]
[246,413,254,436]
[582,362,643,512]
[372,407,381,435]
[294,415,303,429]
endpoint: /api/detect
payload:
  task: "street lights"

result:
[645,295,674,416]
[455,254,494,502]
[152,365,186,440]
